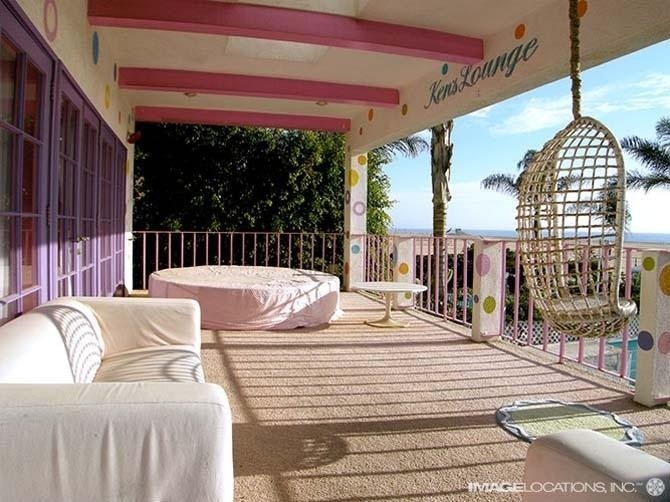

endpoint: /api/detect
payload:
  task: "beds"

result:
[149,265,344,331]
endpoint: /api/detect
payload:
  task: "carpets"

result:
[495,397,646,444]
[200,292,670,502]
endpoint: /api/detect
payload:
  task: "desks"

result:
[351,281,428,328]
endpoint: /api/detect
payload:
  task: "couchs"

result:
[523,432,669,502]
[0,296,235,502]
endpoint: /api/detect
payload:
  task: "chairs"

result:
[514,0,638,339]
